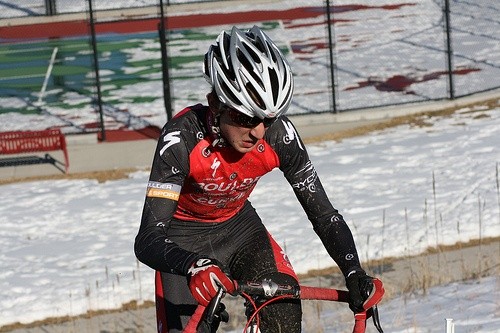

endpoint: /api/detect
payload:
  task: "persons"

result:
[132,24,386,333]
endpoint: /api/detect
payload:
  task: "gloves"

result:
[345,273,386,315]
[185,254,238,305]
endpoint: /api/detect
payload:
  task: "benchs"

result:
[0,129,69,171]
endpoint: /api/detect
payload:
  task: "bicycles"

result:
[180,272,386,333]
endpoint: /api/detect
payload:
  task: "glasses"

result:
[223,105,276,128]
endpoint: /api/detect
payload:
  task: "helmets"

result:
[202,24,294,119]
